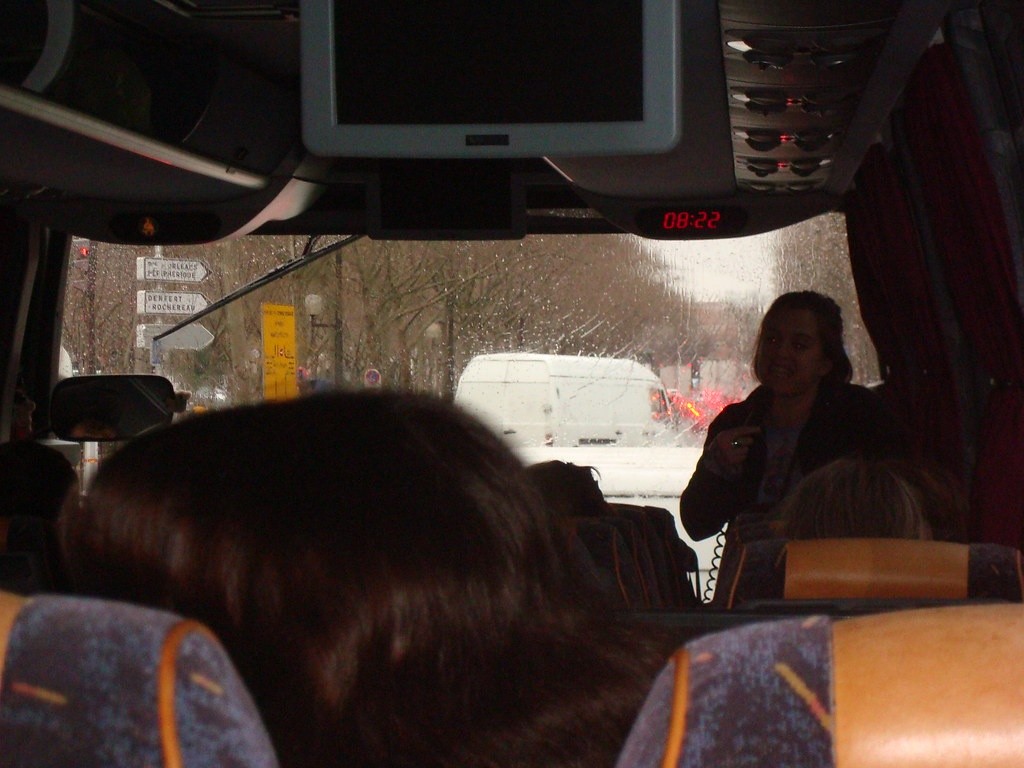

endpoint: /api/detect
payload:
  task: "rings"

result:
[730,439,743,448]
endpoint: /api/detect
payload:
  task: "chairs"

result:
[0,373,281,767]
[551,504,1024,768]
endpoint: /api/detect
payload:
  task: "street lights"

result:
[305,294,344,389]
[425,318,454,399]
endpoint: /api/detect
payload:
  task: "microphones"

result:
[745,387,776,429]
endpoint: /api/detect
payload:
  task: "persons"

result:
[67,415,119,440]
[0,387,668,768]
[680,292,930,610]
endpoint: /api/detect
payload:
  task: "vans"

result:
[454,351,682,450]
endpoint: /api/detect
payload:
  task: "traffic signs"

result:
[135,256,213,284]
[136,324,218,352]
[136,290,214,317]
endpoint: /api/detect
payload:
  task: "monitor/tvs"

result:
[298,0,682,159]
